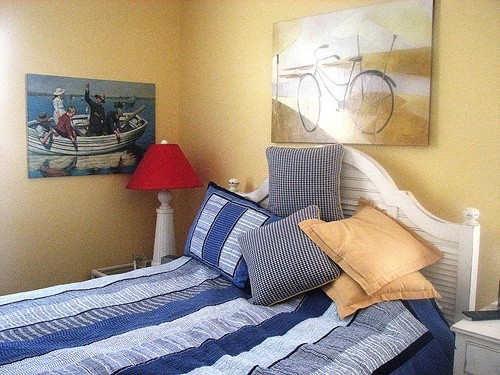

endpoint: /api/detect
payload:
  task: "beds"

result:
[0,142,482,375]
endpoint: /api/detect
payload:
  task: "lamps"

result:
[125,140,203,267]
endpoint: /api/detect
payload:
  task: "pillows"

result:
[320,274,442,319]
[238,208,339,308]
[300,197,441,296]
[180,182,277,291]
[265,145,343,222]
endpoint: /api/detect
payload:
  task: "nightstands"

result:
[450,301,499,375]
[90,254,178,279]
